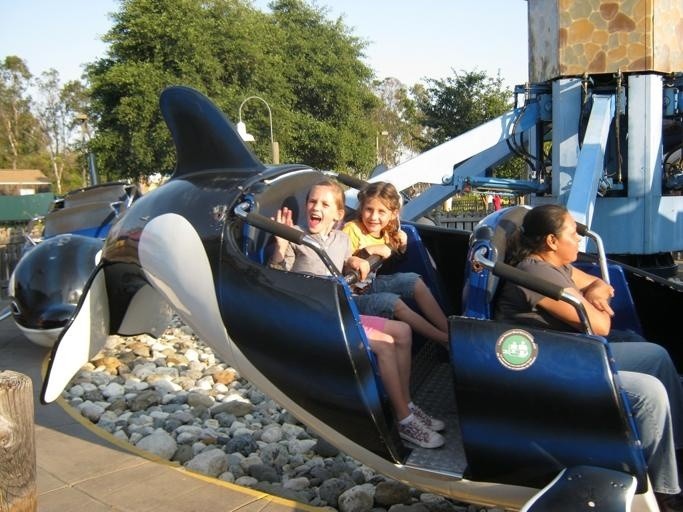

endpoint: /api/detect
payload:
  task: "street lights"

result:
[375,128,389,164]
[236,96,279,165]
[74,114,87,188]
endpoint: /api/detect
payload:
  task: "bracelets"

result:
[270,256,287,268]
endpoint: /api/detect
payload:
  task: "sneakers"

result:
[397,417,445,450]
[406,402,447,431]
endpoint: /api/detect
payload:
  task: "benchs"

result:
[447,205,656,492]
[233,167,450,414]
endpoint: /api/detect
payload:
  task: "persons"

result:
[499,204,682,495]
[268,177,444,449]
[340,182,449,349]
